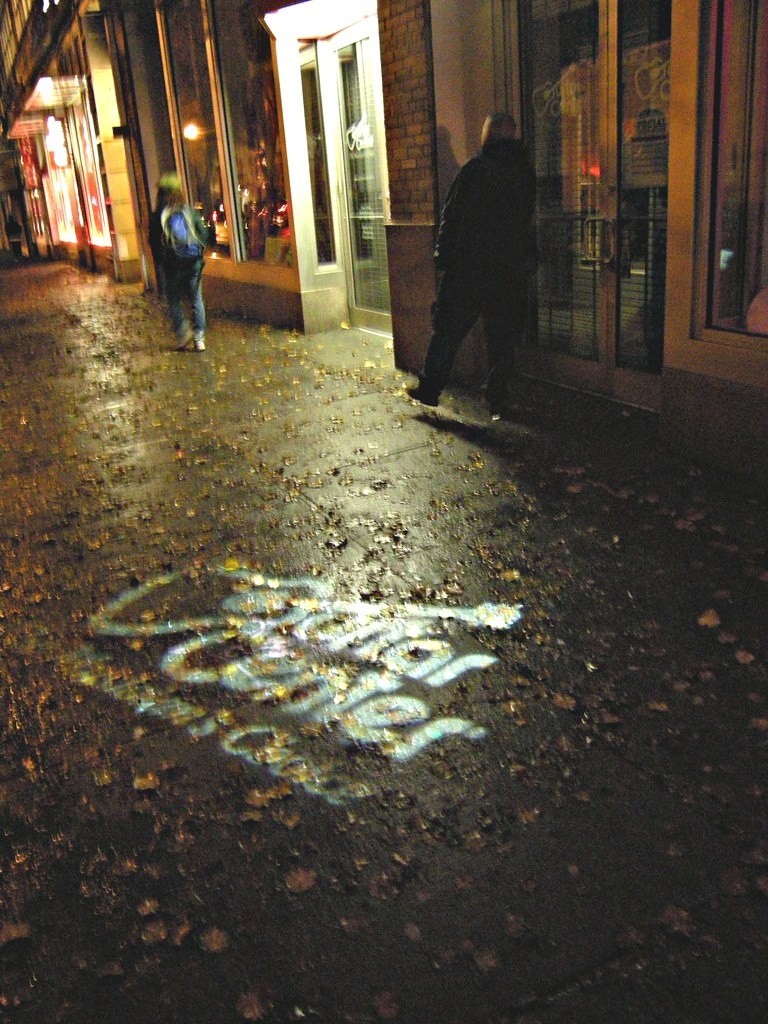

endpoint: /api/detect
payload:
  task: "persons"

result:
[405,113,539,419]
[5,215,23,263]
[148,173,210,352]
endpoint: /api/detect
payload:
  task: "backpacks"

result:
[159,204,203,264]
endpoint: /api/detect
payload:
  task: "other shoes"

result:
[406,386,440,407]
[194,340,206,352]
[174,331,194,350]
